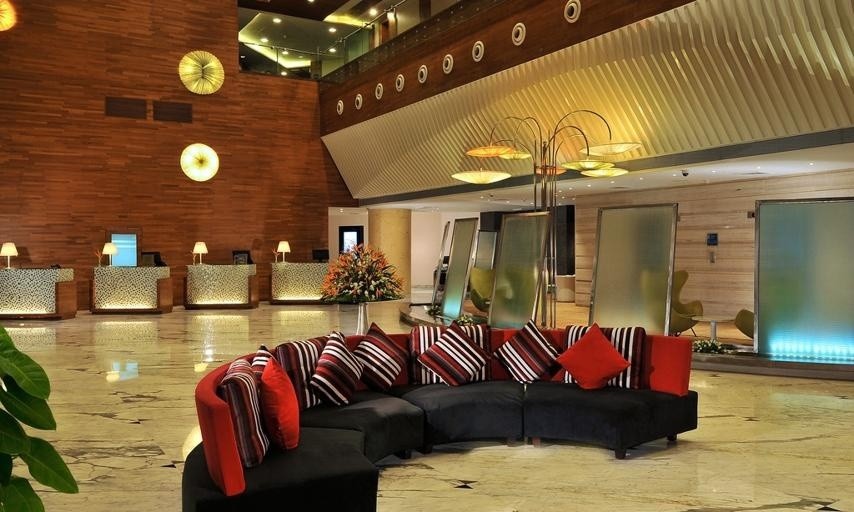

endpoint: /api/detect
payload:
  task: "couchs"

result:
[180,327,700,512]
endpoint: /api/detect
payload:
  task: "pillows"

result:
[349,321,410,395]
[490,317,562,385]
[553,322,631,394]
[415,318,495,388]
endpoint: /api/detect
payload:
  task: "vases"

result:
[355,299,370,337]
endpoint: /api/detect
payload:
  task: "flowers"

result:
[314,238,406,307]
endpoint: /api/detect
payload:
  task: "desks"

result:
[691,312,737,344]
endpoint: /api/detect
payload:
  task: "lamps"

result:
[100,242,119,267]
[276,240,291,264]
[192,241,209,266]
[0,242,18,270]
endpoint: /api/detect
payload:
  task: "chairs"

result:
[733,308,757,342]
[668,266,703,340]
[469,265,494,316]
